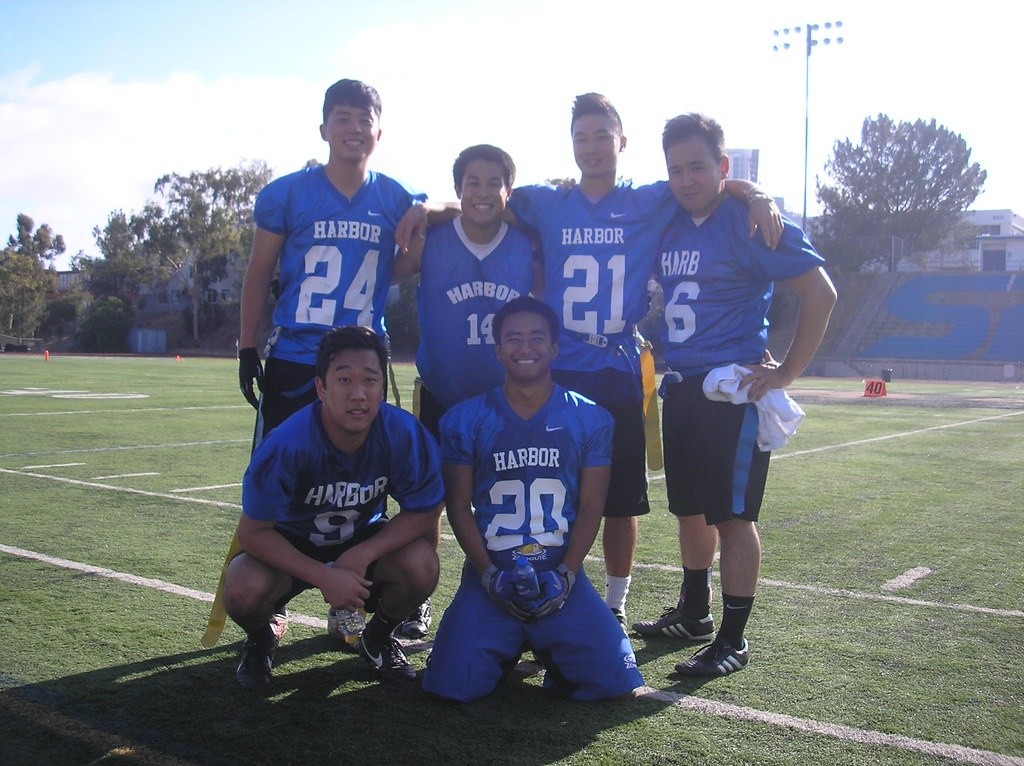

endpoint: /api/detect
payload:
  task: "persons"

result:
[422,298,644,702]
[238,79,839,676]
[221,327,444,688]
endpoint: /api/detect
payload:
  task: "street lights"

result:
[775,21,843,232]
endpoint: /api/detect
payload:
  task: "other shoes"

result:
[609,606,627,632]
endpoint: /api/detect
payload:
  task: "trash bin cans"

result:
[881,368,892,382]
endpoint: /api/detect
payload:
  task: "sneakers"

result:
[358,635,417,681]
[327,605,366,648]
[402,597,432,639]
[675,638,750,676]
[237,636,280,691]
[632,607,716,639]
[269,610,289,639]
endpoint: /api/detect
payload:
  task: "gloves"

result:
[526,564,575,620]
[480,564,539,621]
[238,347,266,411]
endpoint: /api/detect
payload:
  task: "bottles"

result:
[324,559,366,635]
[513,557,540,601]
